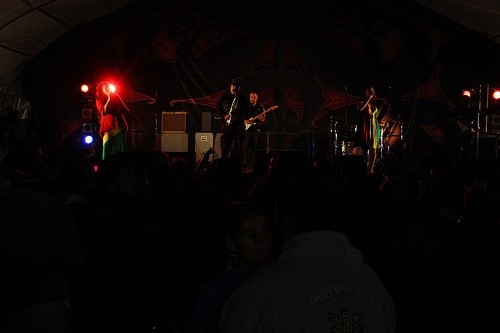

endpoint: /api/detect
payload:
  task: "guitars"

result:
[244,106,279,131]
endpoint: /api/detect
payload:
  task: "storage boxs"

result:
[162,111,188,133]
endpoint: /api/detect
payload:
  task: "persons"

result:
[357,87,382,174]
[240,92,267,174]
[215,77,248,166]
[0,106,500,333]
[96,81,131,180]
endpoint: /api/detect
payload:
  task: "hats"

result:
[229,79,242,85]
